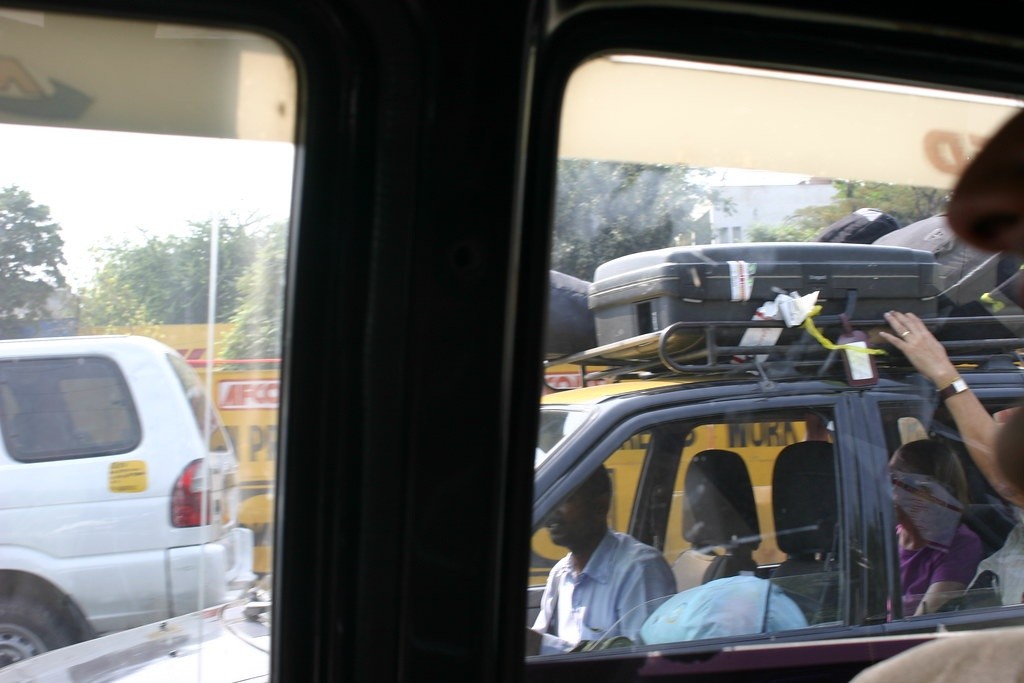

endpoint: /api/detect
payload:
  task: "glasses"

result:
[889,472,937,493]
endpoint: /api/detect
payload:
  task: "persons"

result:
[889,439,1024,615]
[879,309,1024,505]
[526,463,678,656]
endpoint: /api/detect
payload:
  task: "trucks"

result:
[64,319,642,583]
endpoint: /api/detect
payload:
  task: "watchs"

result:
[941,378,969,401]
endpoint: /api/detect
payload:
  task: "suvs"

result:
[1,335,257,666]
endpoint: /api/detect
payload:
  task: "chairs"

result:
[670,449,762,592]
[770,442,840,626]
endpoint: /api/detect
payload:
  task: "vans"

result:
[531,417,810,585]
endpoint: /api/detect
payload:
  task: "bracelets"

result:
[939,376,960,389]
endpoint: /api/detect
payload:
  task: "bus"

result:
[0,312,1024,682]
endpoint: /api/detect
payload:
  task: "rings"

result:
[901,331,911,339]
